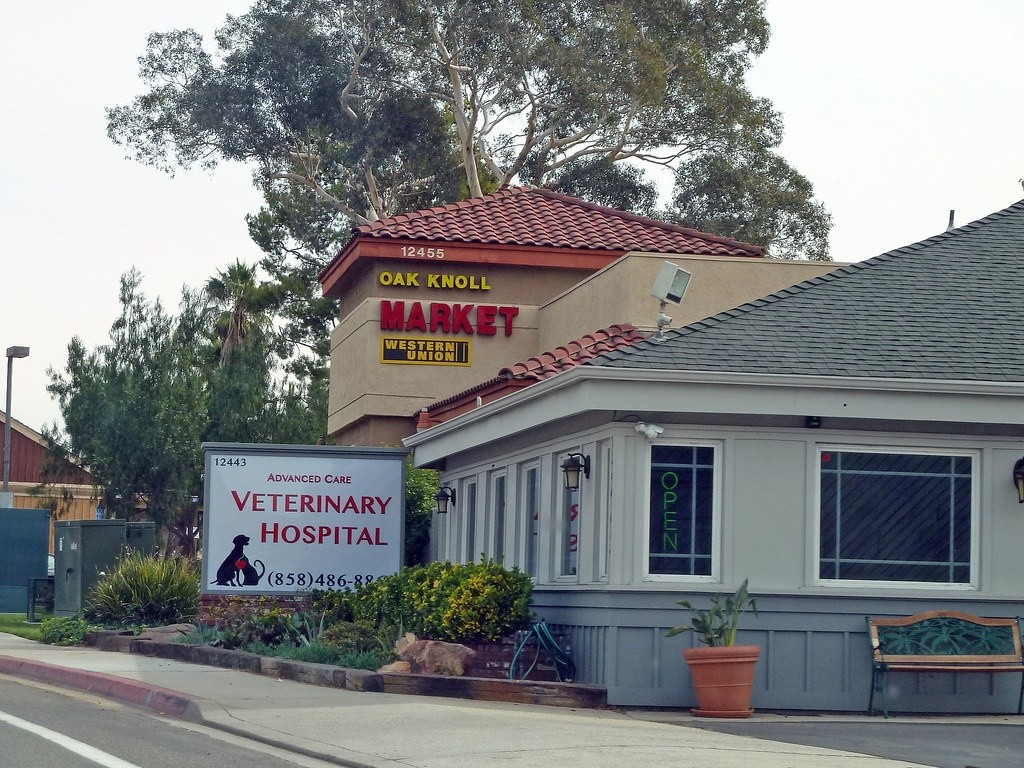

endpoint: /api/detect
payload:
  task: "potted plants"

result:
[665,578,761,719]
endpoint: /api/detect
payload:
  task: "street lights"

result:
[1,346,30,493]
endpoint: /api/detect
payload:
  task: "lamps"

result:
[434,486,456,515]
[651,261,692,306]
[1013,456,1024,503]
[560,452,591,490]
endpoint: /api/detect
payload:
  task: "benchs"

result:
[866,611,1024,719]
